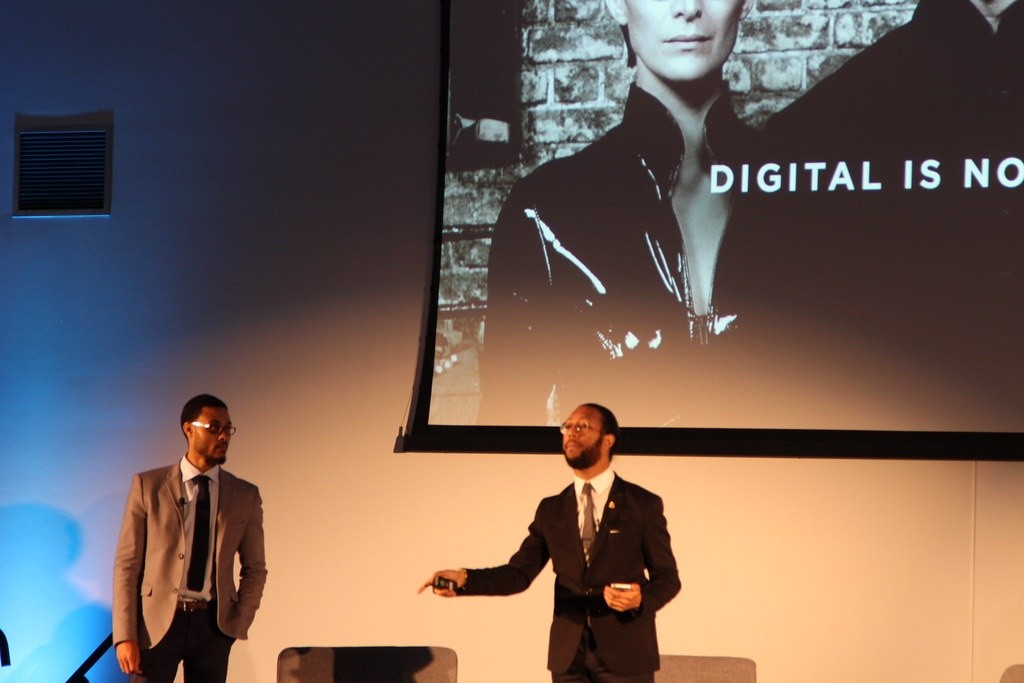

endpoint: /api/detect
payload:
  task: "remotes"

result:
[433,575,457,592]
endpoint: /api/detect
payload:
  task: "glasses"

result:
[191,420,237,436]
[560,418,603,436]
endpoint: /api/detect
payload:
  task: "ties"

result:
[580,483,598,566]
[185,475,212,592]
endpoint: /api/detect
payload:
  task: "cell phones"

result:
[611,583,631,592]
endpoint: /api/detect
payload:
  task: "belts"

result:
[178,600,216,611]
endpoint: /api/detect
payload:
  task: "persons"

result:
[113,393,268,683]
[755,0,1024,434]
[417,403,682,683]
[474,0,757,425]
